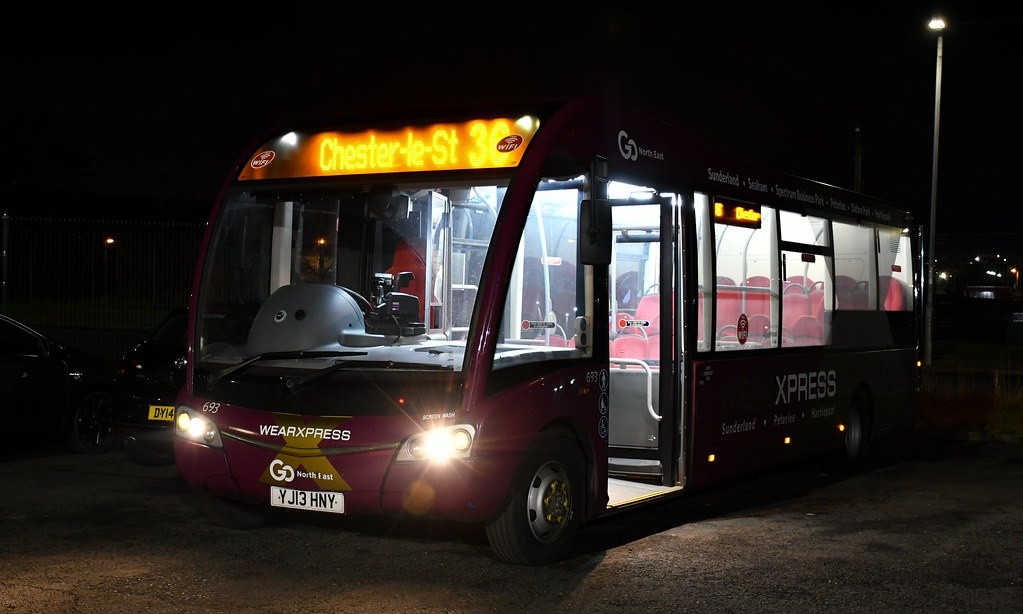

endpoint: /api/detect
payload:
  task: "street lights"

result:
[102,238,115,298]
[922,16,949,369]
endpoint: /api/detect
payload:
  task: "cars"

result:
[0,315,131,456]
[111,311,228,431]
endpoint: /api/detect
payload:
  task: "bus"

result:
[173,93,924,565]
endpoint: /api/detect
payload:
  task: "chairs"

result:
[531,276,905,369]
